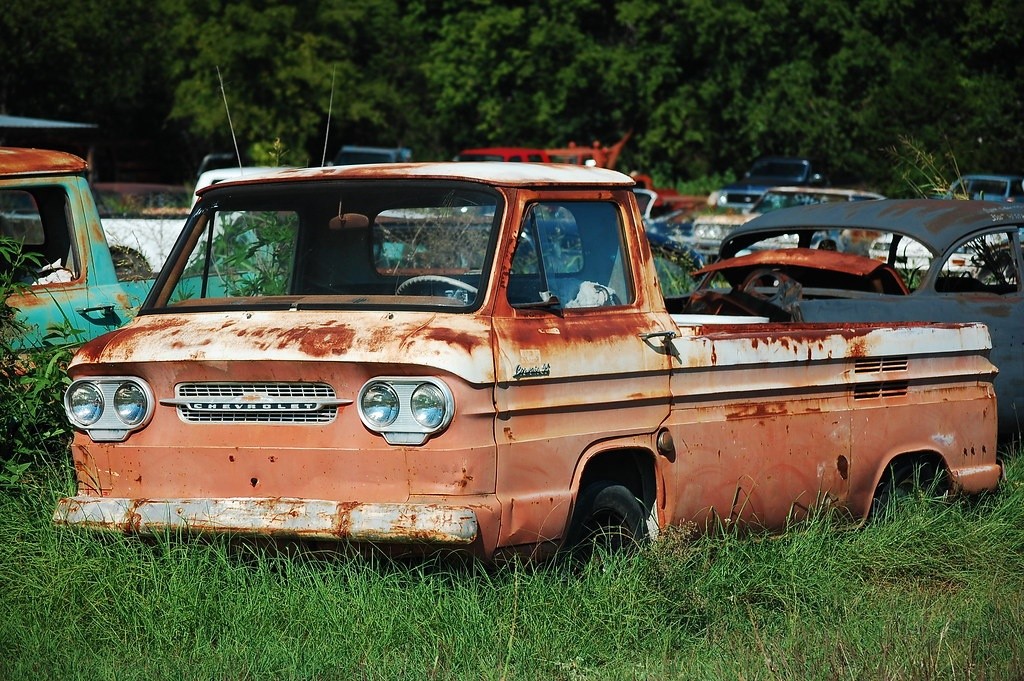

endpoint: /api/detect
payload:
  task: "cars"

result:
[28,112,1024,435]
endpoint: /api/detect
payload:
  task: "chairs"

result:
[309,212,395,293]
[935,278,990,292]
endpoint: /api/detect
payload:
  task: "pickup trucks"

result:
[53,157,1002,576]
[0,146,428,382]
